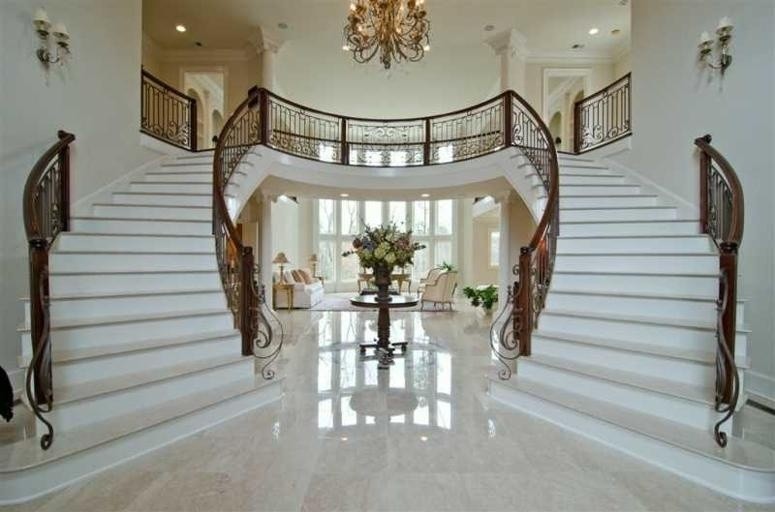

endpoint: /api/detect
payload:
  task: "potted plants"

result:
[462,283,499,310]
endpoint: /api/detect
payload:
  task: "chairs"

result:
[417,266,461,313]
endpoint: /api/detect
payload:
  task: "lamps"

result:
[340,0,432,70]
[273,252,292,285]
[309,254,320,277]
[31,6,72,65]
[696,16,735,74]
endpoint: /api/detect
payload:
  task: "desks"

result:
[272,283,295,313]
[356,272,412,295]
[349,293,420,359]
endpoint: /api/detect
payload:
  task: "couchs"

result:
[272,267,324,309]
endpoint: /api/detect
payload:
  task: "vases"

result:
[372,264,393,301]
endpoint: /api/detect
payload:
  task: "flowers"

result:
[341,216,428,275]
